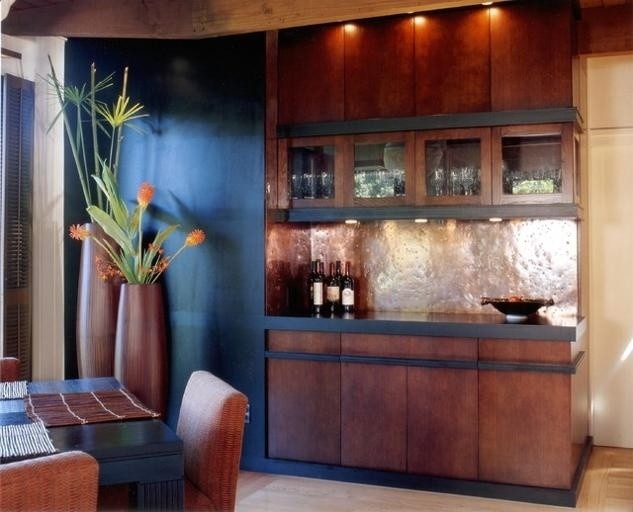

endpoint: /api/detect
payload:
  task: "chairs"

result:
[0,451,98,512]
[95,369,249,512]
[0,355,22,383]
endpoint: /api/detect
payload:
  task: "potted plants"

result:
[38,50,147,381]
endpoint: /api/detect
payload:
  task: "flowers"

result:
[67,153,206,285]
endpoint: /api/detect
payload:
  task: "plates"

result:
[480,297,552,315]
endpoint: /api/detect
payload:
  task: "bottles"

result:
[321,262,325,279]
[337,261,341,286]
[310,261,315,312]
[313,258,324,314]
[326,263,340,314]
[342,262,355,315]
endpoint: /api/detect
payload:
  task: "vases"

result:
[114,284,164,418]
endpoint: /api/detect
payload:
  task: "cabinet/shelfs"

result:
[340,310,479,494]
[277,12,414,126]
[414,0,573,116]
[276,128,414,209]
[267,311,340,478]
[479,312,594,508]
[413,122,577,207]
